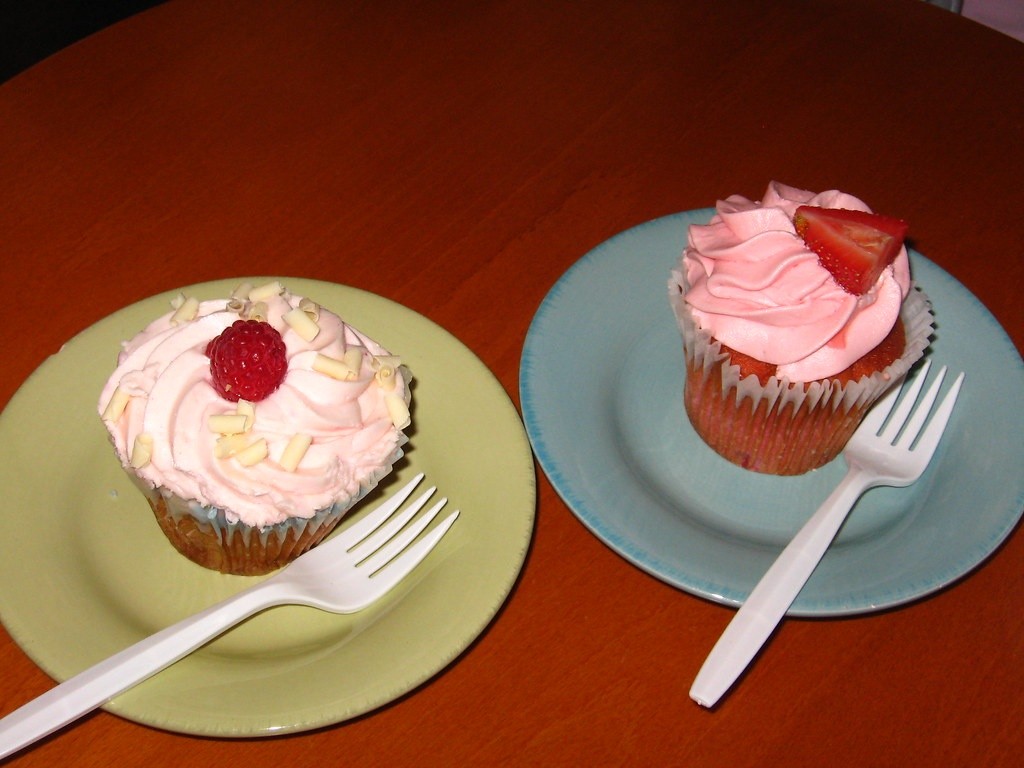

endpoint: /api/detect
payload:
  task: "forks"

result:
[688,359,965,712]
[0,472,462,761]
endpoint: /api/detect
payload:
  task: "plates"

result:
[0,277,538,737]
[517,203,1024,619]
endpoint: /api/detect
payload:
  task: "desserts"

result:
[679,182,912,471]
[99,282,413,575]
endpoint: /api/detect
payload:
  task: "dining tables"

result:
[0,0,1022,767]
